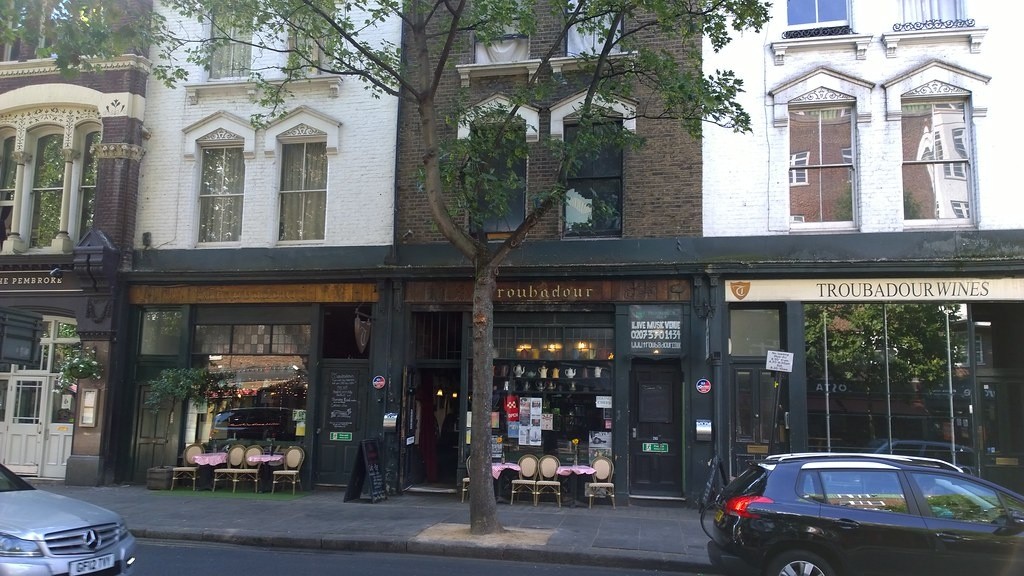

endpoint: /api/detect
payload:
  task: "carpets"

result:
[148,487,319,501]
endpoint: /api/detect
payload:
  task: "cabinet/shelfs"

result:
[493,375,609,442]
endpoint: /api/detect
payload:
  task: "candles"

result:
[292,364,298,370]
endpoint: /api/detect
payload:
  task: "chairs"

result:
[212,445,265,494]
[270,446,305,495]
[461,456,471,502]
[171,444,205,492]
[511,454,536,506]
[534,455,562,507]
[588,456,616,510]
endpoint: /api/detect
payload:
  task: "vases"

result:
[502,446,506,463]
[573,454,578,467]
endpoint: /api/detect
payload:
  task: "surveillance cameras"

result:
[293,365,300,371]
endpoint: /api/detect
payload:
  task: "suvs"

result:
[708,452,1023,576]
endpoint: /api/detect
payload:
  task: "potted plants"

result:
[145,367,210,490]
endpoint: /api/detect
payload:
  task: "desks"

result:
[492,462,520,504]
[191,452,228,491]
[554,465,597,508]
[247,454,284,494]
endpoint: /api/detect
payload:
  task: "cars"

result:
[860,438,986,478]
[210,407,297,440]
[0,463,139,576]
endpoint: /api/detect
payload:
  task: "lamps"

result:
[402,229,416,245]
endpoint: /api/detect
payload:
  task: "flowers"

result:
[498,437,504,446]
[572,438,578,462]
[51,347,105,394]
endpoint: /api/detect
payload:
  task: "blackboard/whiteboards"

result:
[326,369,359,432]
[700,454,728,508]
[342,438,389,503]
